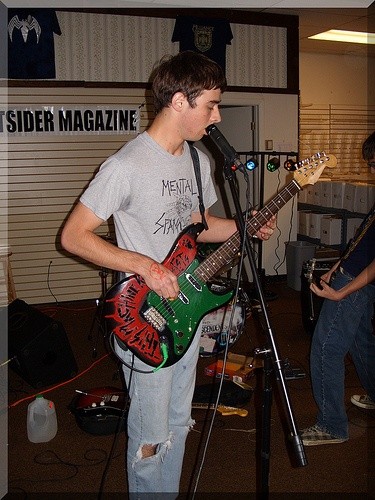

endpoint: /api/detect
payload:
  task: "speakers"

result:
[0,298,79,389]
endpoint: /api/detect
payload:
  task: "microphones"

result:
[206,125,248,176]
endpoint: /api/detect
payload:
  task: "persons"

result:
[298,133,375,446]
[62,50,277,500]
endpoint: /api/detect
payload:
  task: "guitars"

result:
[96,151,338,368]
[71,387,252,429]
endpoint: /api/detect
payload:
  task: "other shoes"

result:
[350,394,375,410]
[290,423,349,446]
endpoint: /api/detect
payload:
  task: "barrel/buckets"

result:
[27,394,58,444]
